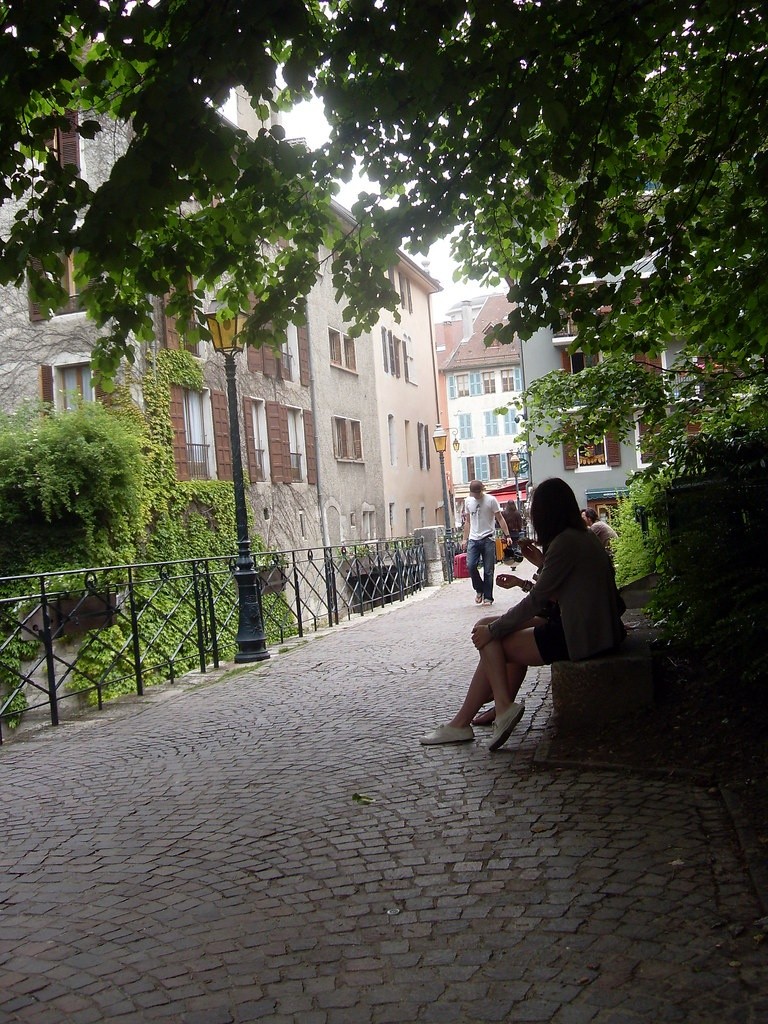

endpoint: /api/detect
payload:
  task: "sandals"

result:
[473,706,496,724]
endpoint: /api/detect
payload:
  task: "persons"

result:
[470,538,559,724]
[418,477,625,751]
[461,480,512,607]
[580,508,618,557]
[500,499,523,559]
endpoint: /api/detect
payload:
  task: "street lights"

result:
[510,453,521,511]
[205,288,272,663]
[432,424,452,537]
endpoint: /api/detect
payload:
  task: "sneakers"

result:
[489,703,525,751]
[419,723,473,744]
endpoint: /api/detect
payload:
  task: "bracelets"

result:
[506,535,511,538]
[522,579,532,593]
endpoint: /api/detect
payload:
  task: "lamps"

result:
[444,427,459,451]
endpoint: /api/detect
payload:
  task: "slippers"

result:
[476,591,492,607]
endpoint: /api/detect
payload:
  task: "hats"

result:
[470,480,485,492]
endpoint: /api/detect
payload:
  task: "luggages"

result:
[495,538,507,561]
[454,553,470,578]
